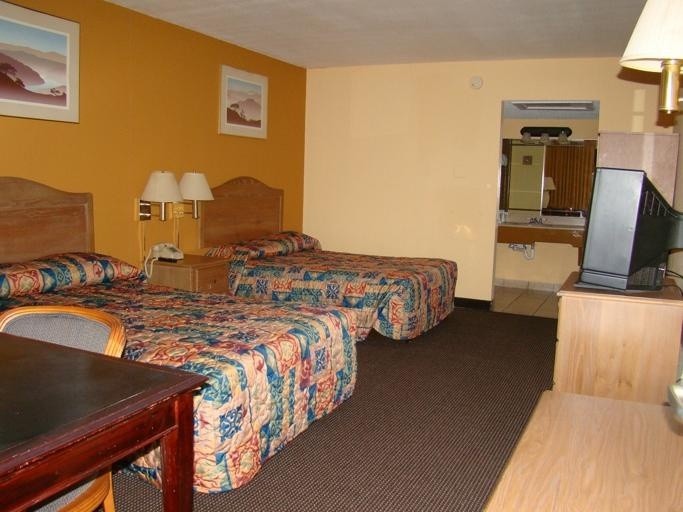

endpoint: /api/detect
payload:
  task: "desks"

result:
[1,330,210,511]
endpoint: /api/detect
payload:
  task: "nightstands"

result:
[146,253,229,296]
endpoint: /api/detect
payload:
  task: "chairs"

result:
[0,304,128,512]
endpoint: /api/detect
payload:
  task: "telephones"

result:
[144,243,183,278]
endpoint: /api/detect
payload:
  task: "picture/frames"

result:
[217,62,269,141]
[1,0,82,125]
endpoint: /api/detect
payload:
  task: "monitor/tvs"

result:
[573,164,683,295]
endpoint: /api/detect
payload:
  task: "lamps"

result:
[557,132,569,144]
[538,133,553,145]
[618,0,683,116]
[177,171,215,219]
[520,132,534,144]
[138,168,184,222]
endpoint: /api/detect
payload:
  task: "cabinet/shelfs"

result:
[553,270,681,406]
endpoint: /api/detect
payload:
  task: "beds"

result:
[0,176,357,495]
[198,175,458,342]
